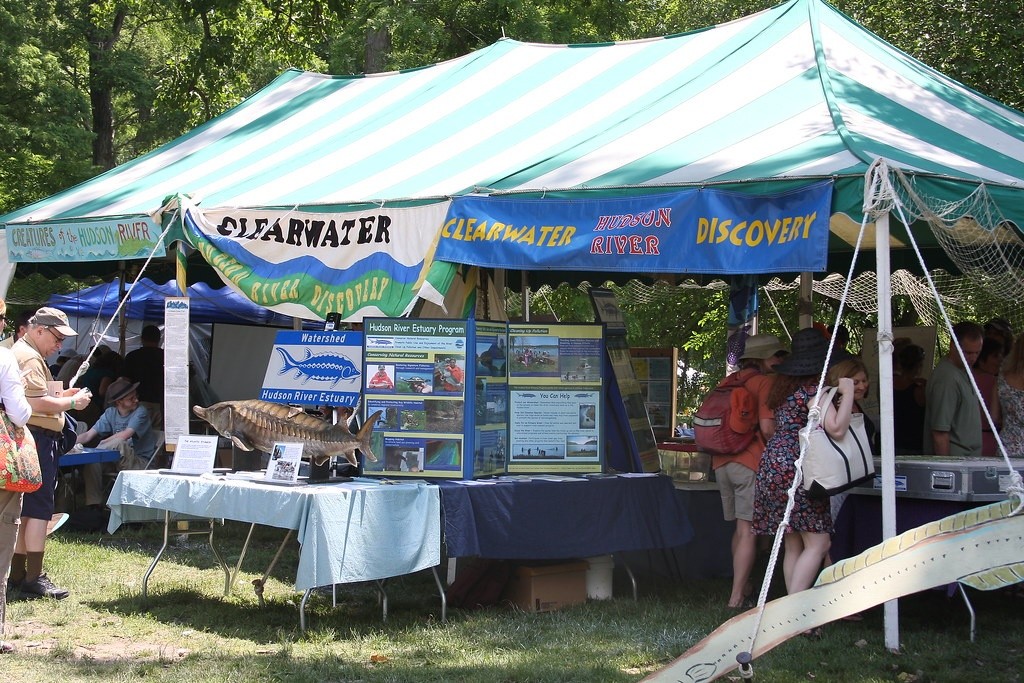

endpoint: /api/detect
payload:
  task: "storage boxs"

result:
[656,443,712,482]
[843,456,1024,502]
[510,561,588,612]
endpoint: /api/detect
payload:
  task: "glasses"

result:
[45,326,65,345]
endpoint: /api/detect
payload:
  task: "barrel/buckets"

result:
[584,554,615,600]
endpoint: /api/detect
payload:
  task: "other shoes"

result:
[0,641,15,654]
[802,629,820,638]
[843,613,865,622]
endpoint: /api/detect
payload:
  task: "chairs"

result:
[126,431,164,472]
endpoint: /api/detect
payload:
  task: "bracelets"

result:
[71,397,75,409]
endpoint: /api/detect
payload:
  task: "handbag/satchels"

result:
[0,404,42,493]
[798,384,877,500]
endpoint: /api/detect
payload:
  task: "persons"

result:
[712,333,791,608]
[0,299,93,654]
[370,365,393,389]
[751,326,831,637]
[41,325,222,435]
[822,360,875,622]
[877,318,1024,459]
[487,432,504,470]
[440,358,464,392]
[827,324,858,368]
[70,375,159,531]
[521,343,551,367]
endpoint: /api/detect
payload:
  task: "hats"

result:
[772,327,851,376]
[106,376,141,405]
[34,306,78,336]
[892,337,925,366]
[60,348,77,358]
[737,334,791,359]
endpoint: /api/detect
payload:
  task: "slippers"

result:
[725,596,754,614]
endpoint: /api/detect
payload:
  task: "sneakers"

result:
[8,571,28,589]
[22,572,70,599]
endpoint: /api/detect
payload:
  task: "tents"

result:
[45,275,325,373]
[0,1,1024,655]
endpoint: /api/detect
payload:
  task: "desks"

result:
[669,480,734,579]
[57,446,121,534]
[830,488,1018,644]
[109,462,447,639]
[424,472,690,603]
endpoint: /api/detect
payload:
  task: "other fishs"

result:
[439,364,458,387]
[193,399,383,467]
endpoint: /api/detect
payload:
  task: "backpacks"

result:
[693,371,767,453]
